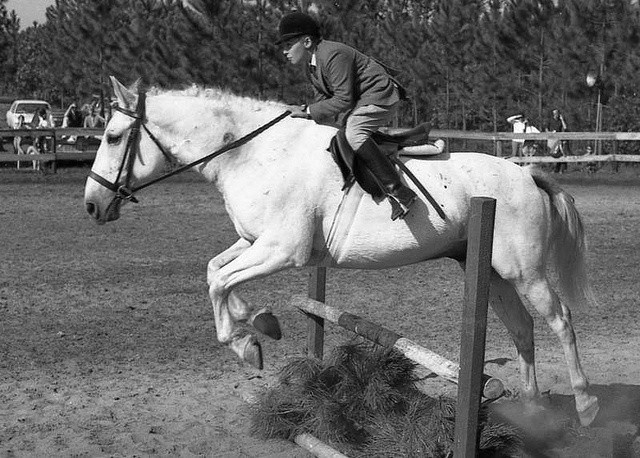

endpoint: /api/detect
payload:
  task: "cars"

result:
[6,100,55,131]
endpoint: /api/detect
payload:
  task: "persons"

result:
[276,12,416,220]
[582,146,599,173]
[36,110,55,151]
[14,117,30,150]
[507,113,525,165]
[546,109,567,174]
[85,108,104,138]
[60,104,83,142]
[523,119,540,165]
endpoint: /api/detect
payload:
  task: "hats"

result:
[273,13,320,44]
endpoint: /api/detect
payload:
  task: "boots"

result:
[358,137,418,220]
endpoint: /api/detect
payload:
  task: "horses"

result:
[85,74,601,430]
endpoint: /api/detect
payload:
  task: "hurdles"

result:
[242,196,503,458]
